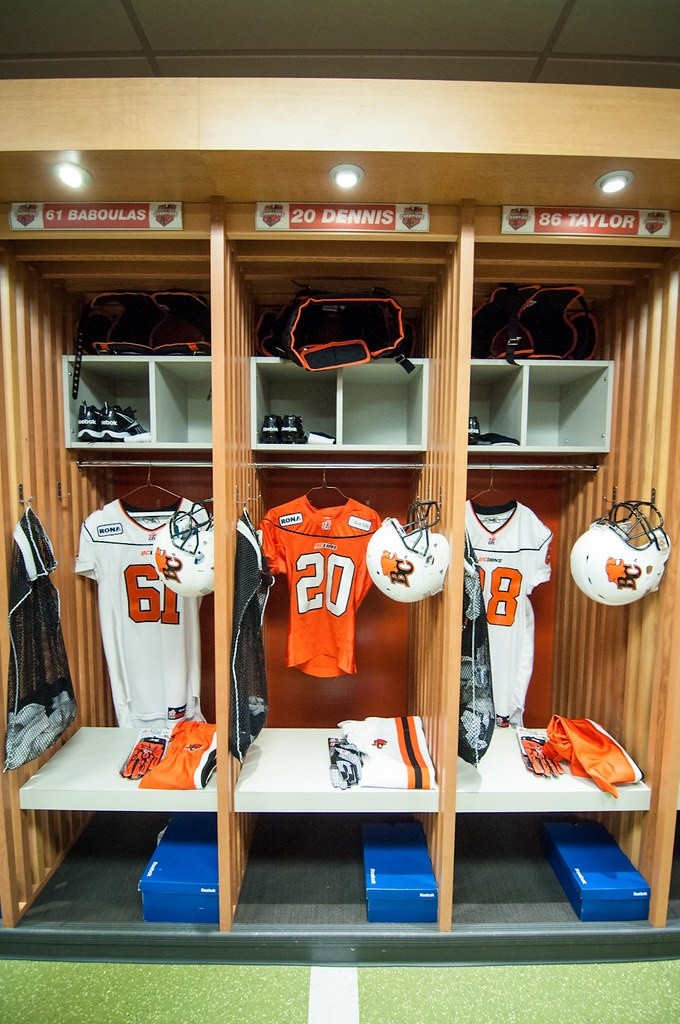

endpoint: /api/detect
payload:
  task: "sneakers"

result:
[469,417,481,444]
[262,415,283,443]
[280,415,307,443]
[99,401,148,440]
[77,401,101,442]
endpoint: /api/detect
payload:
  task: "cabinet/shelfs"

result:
[251,357,428,452]
[468,358,615,453]
[61,354,213,450]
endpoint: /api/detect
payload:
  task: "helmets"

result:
[366,501,450,602]
[570,499,672,605]
[153,501,216,598]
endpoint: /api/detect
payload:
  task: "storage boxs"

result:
[543,820,651,920]
[137,813,219,922]
[362,821,437,922]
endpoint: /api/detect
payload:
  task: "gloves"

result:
[515,728,564,778]
[119,730,163,780]
[326,735,362,789]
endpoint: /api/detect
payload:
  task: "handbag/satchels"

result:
[473,282,599,363]
[78,287,213,359]
[255,290,418,373]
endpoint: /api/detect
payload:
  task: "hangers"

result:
[471,462,516,502]
[306,462,351,500]
[120,460,181,500]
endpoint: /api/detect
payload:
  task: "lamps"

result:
[600,175,628,193]
[334,170,359,188]
